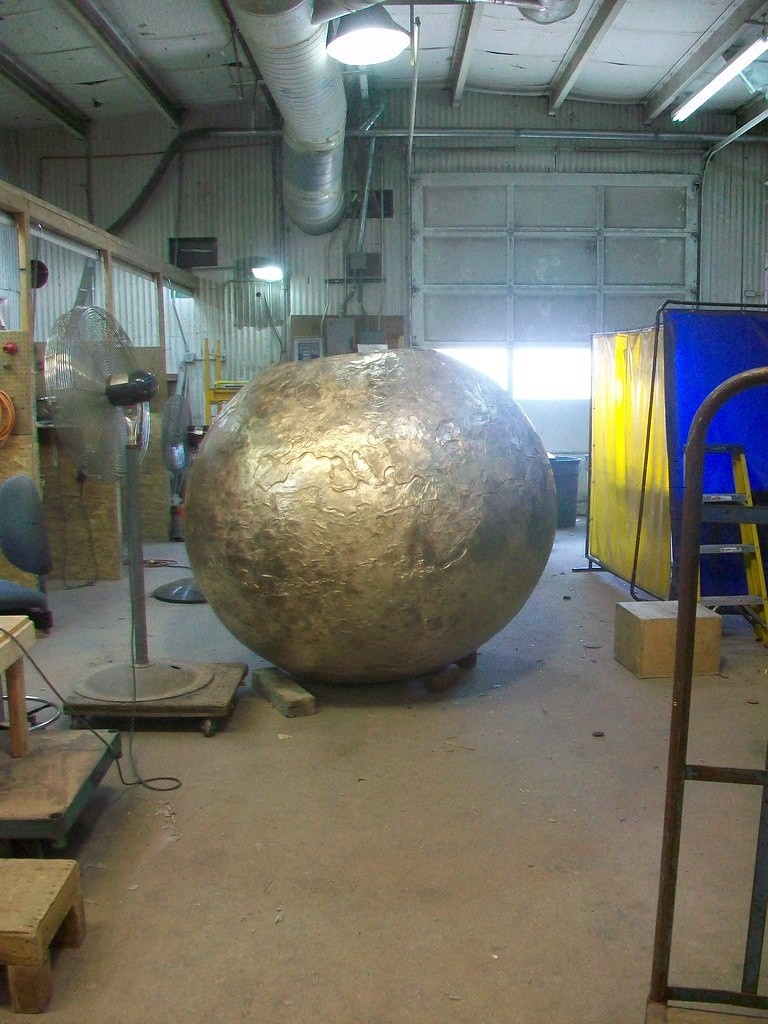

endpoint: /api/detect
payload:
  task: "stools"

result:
[0,857,85,1014]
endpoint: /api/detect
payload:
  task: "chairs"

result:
[204,337,241,426]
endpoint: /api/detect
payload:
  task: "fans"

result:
[153,393,211,605]
[40,306,216,706]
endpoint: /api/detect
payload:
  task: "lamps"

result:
[309,0,386,26]
[249,256,283,282]
[324,3,412,67]
[670,27,768,122]
